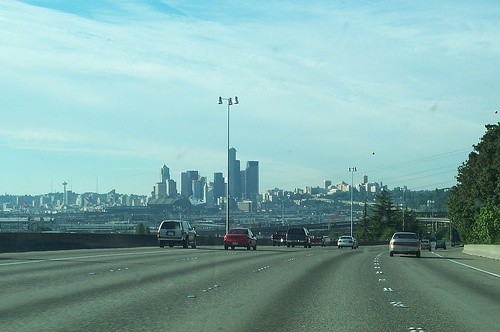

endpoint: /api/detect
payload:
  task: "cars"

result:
[337,236,359,249]
[223,227,258,250]
[388,231,422,257]
[272,226,328,248]
[420,239,431,251]
[435,240,447,250]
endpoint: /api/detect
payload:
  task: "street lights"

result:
[399,187,405,232]
[216,95,241,235]
[427,199,435,233]
[347,165,358,236]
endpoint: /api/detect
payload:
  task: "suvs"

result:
[156,219,199,249]
